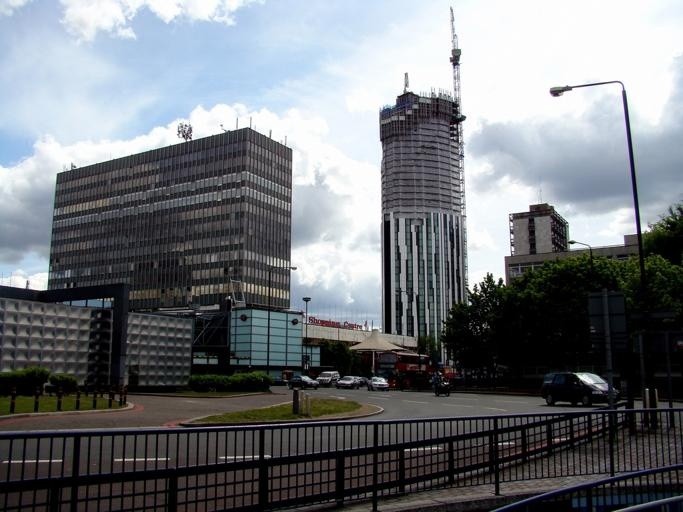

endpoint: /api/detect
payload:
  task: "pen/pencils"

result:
[542,372,621,406]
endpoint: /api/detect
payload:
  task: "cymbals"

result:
[449,6,468,305]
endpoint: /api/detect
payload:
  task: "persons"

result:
[432,371,447,396]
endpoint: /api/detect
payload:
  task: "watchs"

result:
[430,375,450,396]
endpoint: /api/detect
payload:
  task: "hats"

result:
[288,371,390,391]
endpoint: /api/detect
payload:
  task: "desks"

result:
[303,297,311,371]
[266,266,297,377]
[550,80,658,432]
[567,239,593,268]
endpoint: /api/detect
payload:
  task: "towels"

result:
[645,389,659,422]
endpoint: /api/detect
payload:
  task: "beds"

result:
[349,330,408,377]
